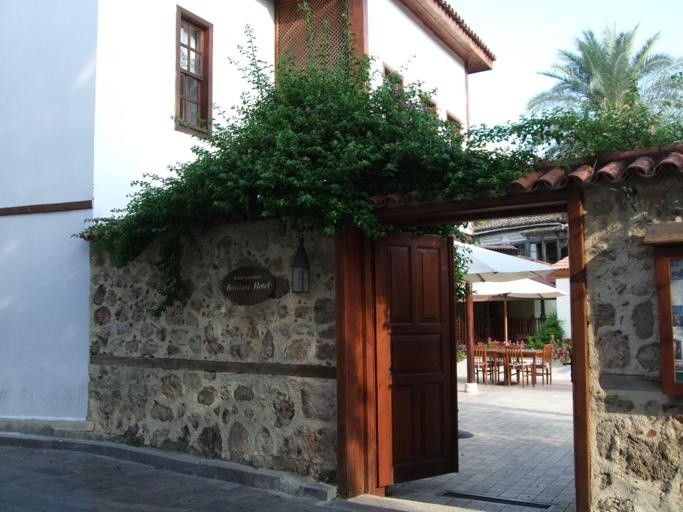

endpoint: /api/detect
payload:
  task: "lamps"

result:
[291,238,310,294]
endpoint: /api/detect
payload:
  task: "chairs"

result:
[473,343,553,388]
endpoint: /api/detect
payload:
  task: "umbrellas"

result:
[452,278,567,319]
[453,238,559,286]
